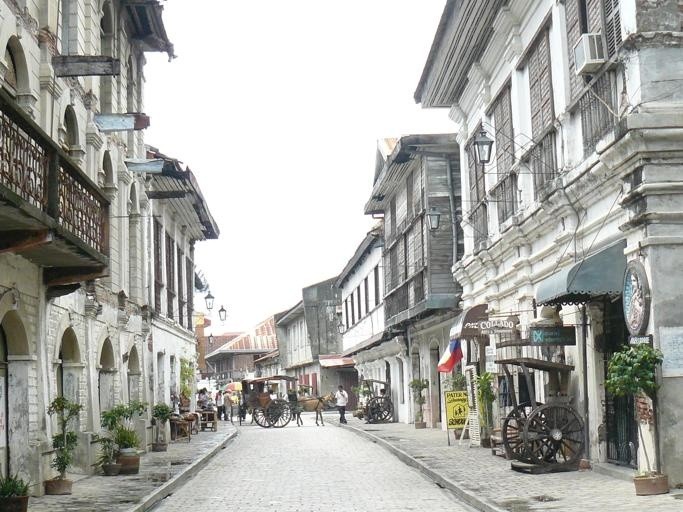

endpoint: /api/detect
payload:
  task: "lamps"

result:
[218,305,226,320]
[337,321,344,334]
[168,290,214,318]
[427,203,488,238]
[208,333,213,343]
[474,122,560,174]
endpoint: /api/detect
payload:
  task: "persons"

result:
[378,388,387,406]
[195,387,245,421]
[334,385,348,423]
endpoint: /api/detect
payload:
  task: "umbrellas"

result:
[219,381,242,391]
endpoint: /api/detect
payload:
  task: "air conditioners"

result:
[572,34,604,75]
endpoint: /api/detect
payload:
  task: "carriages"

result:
[240,375,337,428]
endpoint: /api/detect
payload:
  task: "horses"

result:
[297,391,337,427]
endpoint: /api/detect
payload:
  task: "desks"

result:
[195,411,217,432]
[488,431,520,458]
[170,421,192,442]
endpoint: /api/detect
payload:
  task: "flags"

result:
[433,339,463,372]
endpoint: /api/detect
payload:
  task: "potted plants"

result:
[407,379,428,429]
[90,434,122,475]
[45,396,83,495]
[443,374,469,439]
[605,342,669,496]
[472,372,496,448]
[351,386,362,417]
[0,464,30,512]
[151,403,170,452]
[179,358,195,413]
[101,399,148,474]
